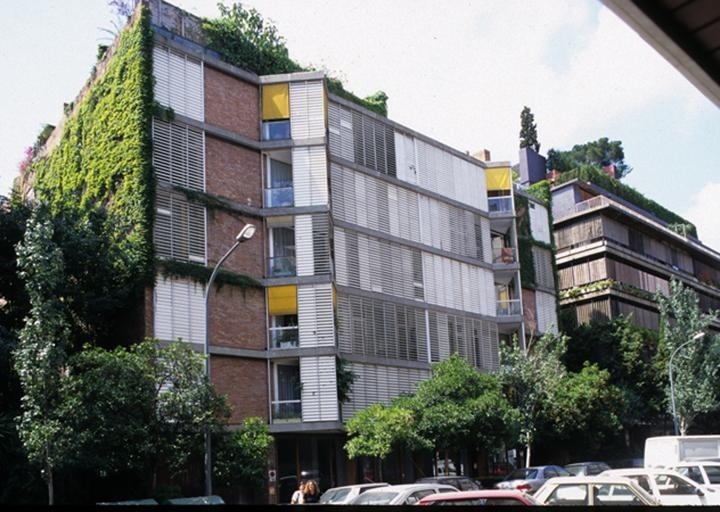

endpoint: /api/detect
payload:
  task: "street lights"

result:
[668,332,707,435]
[202,223,258,505]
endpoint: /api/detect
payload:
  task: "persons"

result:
[303,480,323,503]
[291,481,305,505]
